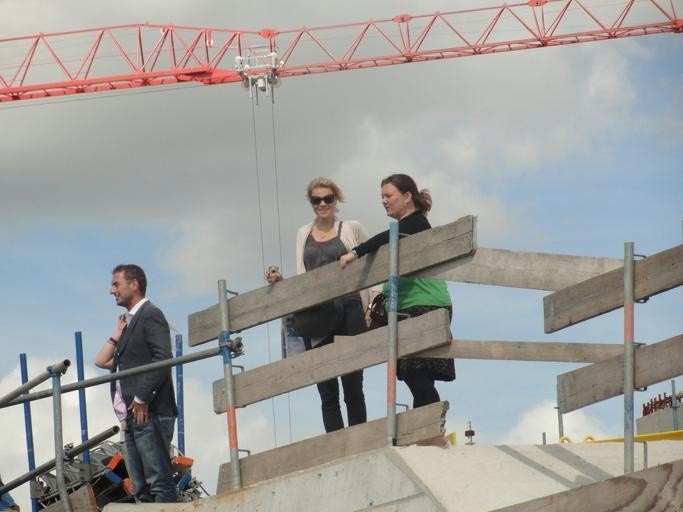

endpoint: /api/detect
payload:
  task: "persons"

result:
[95,315,132,478]
[262,177,376,436]
[106,264,184,503]
[339,173,456,412]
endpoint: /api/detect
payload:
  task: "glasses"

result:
[311,195,335,205]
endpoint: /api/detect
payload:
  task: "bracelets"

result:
[351,249,357,257]
[107,337,117,345]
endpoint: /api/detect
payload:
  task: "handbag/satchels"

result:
[293,295,345,337]
[372,293,387,328]
